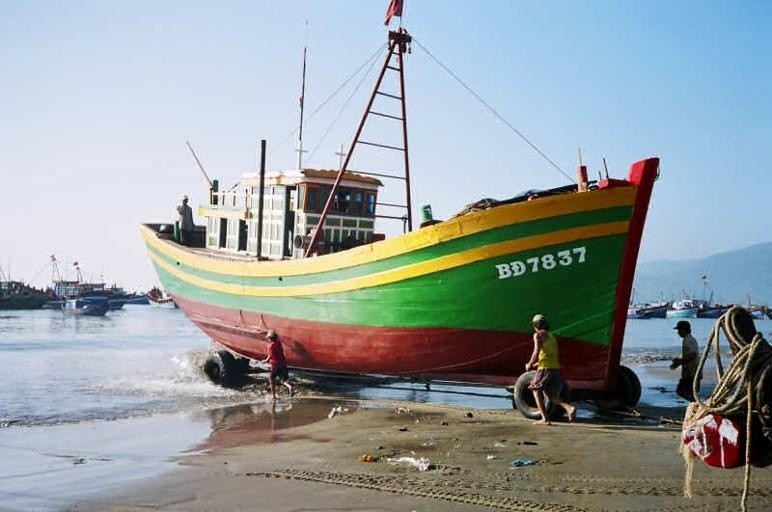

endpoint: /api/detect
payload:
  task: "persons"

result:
[176,195,195,245]
[257,329,294,399]
[524,315,576,424]
[670,320,703,404]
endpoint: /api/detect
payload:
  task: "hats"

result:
[182,195,188,201]
[531,314,545,322]
[673,320,690,330]
[265,331,278,339]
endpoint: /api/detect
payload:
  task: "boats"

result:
[137,3,675,419]
[0,253,177,317]
[624,295,772,322]
